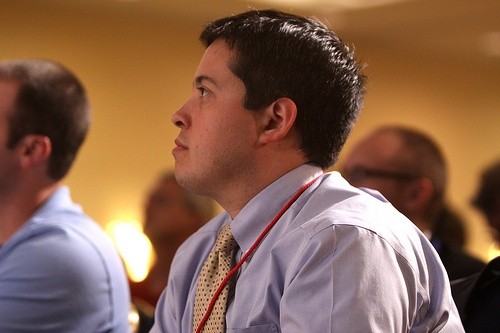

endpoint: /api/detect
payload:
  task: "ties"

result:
[193,224,237,333]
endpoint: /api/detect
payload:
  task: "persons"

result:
[337,122,500,333]
[128,169,219,333]
[0,57,130,333]
[144,8,468,333]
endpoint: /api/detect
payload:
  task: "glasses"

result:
[341,165,419,197]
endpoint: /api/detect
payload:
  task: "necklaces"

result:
[196,173,324,333]
